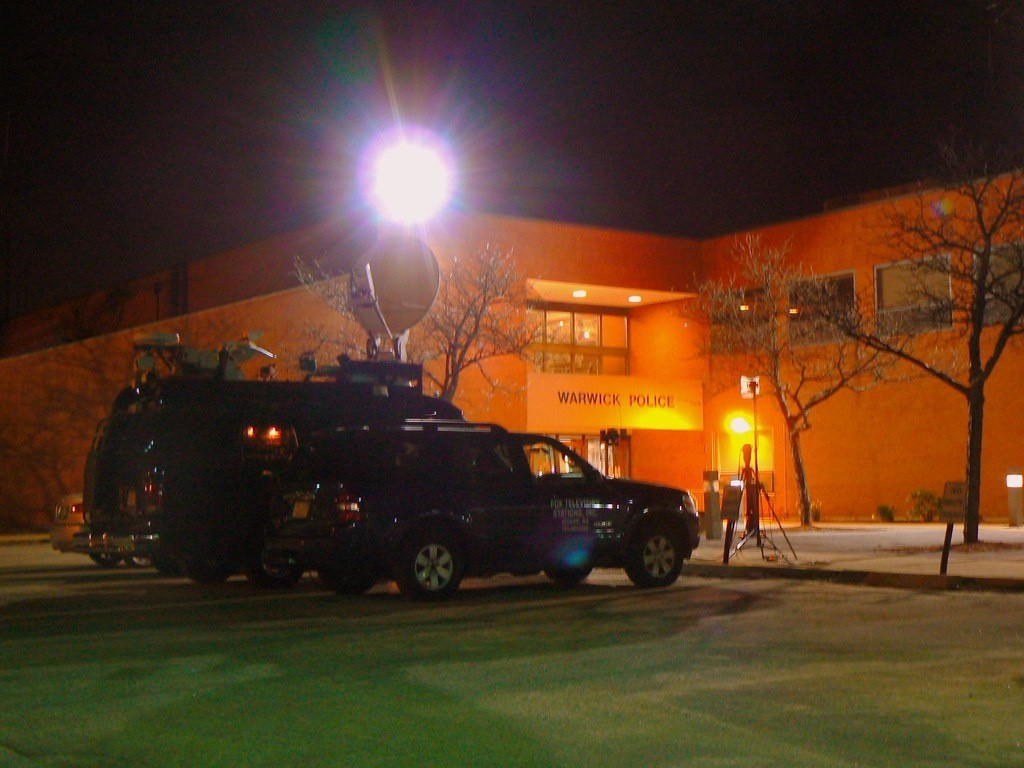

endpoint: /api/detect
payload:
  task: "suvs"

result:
[240,411,701,603]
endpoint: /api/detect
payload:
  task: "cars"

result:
[49,493,153,568]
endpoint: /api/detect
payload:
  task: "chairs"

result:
[476,449,510,474]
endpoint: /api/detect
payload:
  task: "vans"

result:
[72,323,511,587]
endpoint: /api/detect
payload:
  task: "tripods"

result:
[729,381,795,566]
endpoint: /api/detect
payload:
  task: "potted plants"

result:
[877,504,897,522]
[810,500,824,521]
[906,490,955,524]
